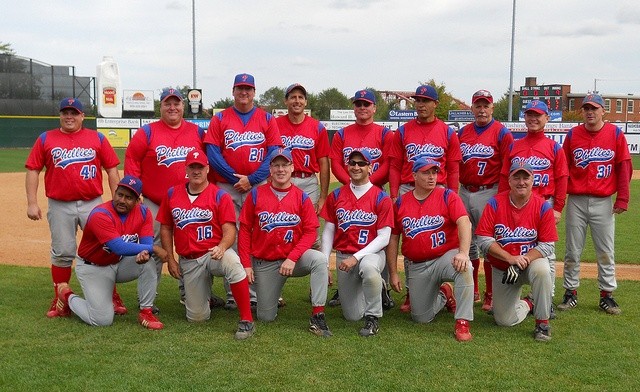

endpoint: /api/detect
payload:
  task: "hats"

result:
[353,90,375,104]
[160,89,182,102]
[581,94,604,108]
[234,74,254,88]
[411,85,438,101]
[412,158,439,172]
[472,90,493,104]
[286,83,306,94]
[348,149,371,161]
[185,149,208,167]
[270,149,292,162]
[117,175,143,197]
[510,162,532,176]
[524,100,548,114]
[59,97,83,113]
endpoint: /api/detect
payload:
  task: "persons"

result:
[203,74,285,311]
[458,89,515,311]
[274,83,331,304]
[386,155,472,342]
[155,148,255,341]
[237,147,334,337]
[331,89,395,310]
[557,94,633,315]
[319,149,396,337]
[55,176,169,330]
[124,88,216,306]
[498,99,570,318]
[388,85,462,313]
[23,97,127,318]
[473,160,559,342]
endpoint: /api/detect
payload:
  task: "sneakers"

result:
[250,298,257,309]
[400,295,410,313]
[557,291,578,312]
[47,298,58,318]
[534,323,551,342]
[454,319,472,342]
[309,312,333,338]
[113,292,127,314]
[383,293,393,309]
[225,298,237,311]
[235,320,255,340]
[439,282,456,314]
[56,282,73,318]
[329,290,339,307]
[360,316,380,336]
[481,292,493,311]
[599,293,621,315]
[139,309,163,330]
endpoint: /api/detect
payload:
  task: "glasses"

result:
[473,91,491,97]
[349,160,369,166]
[271,164,292,169]
[355,101,371,107]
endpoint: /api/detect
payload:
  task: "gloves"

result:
[502,264,520,285]
